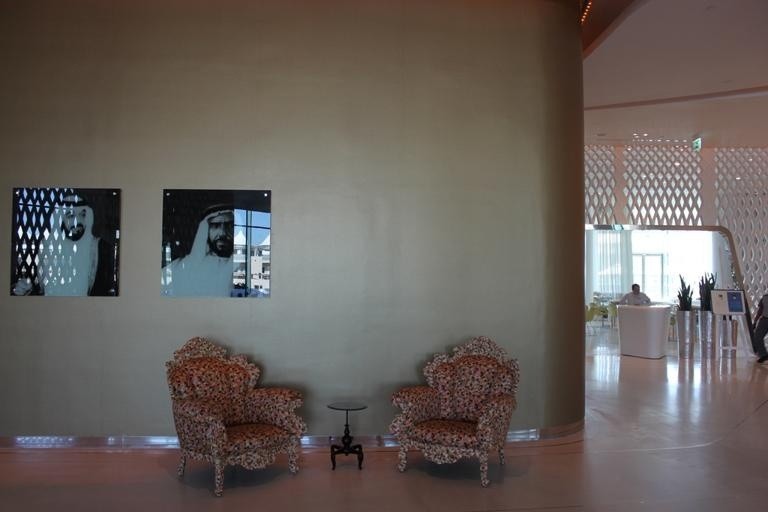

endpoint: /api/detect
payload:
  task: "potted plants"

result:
[698,272,718,359]
[676,274,695,360]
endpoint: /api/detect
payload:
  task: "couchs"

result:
[160,336,308,496]
[385,333,522,489]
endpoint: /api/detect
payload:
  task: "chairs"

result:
[585,298,618,336]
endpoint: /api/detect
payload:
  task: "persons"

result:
[751,291,767,361]
[620,283,650,304]
[14,194,117,296]
[161,200,234,294]
